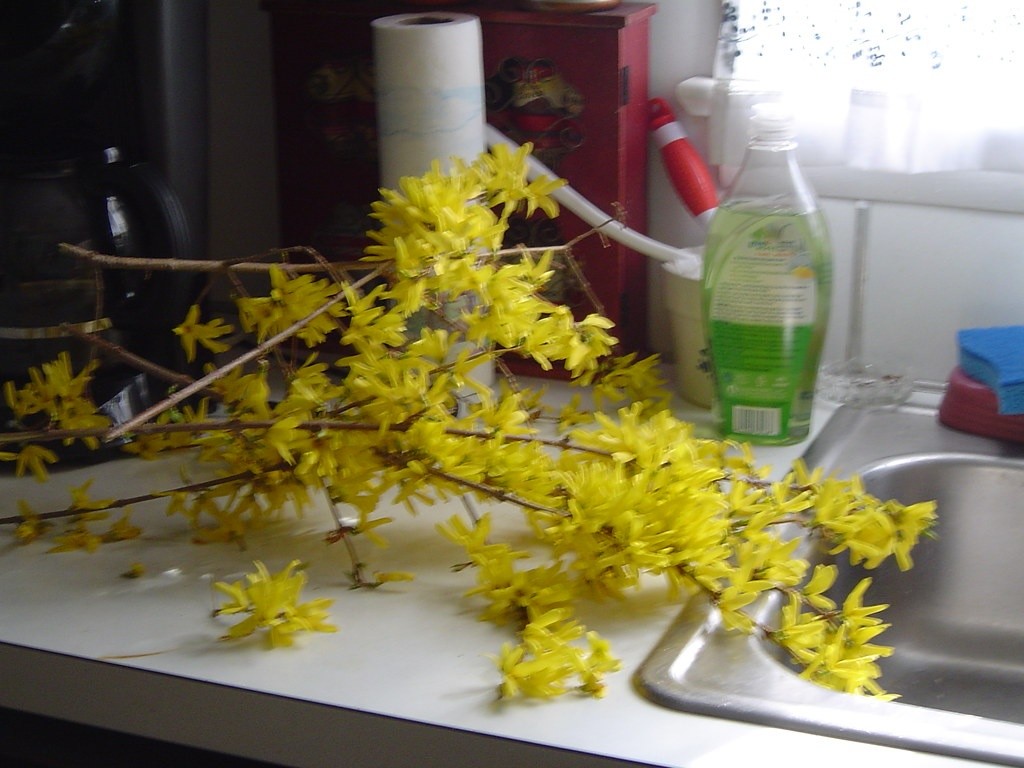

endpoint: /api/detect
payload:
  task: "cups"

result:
[660,245,723,410]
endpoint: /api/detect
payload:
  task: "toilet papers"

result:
[371,13,494,418]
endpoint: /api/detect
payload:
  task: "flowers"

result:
[0,141,939,702]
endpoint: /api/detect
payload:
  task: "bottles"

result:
[703,104,830,446]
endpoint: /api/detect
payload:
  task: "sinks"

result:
[631,404,1024,768]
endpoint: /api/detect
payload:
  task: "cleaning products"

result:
[699,99,831,447]
